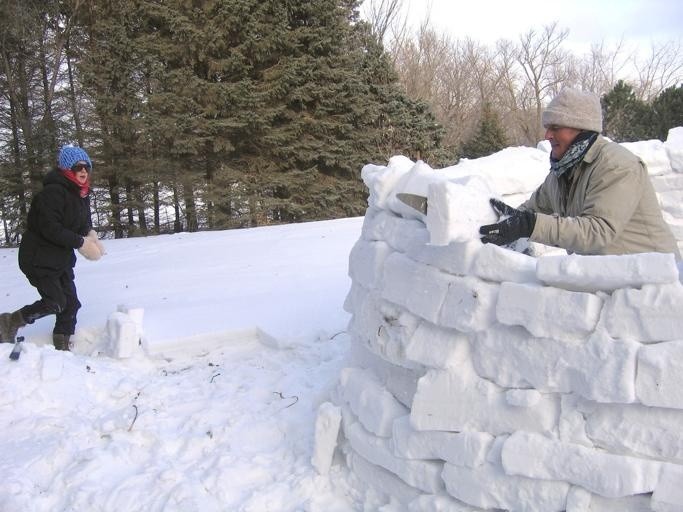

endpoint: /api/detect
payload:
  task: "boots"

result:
[53,333,71,350]
[0,310,27,343]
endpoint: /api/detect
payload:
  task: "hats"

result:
[58,144,93,172]
[541,87,604,134]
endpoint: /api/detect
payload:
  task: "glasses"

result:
[73,164,91,173]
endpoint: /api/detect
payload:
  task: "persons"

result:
[476,87,683,285]
[0,143,107,350]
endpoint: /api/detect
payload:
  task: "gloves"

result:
[78,236,101,260]
[478,198,535,246]
[87,230,103,256]
